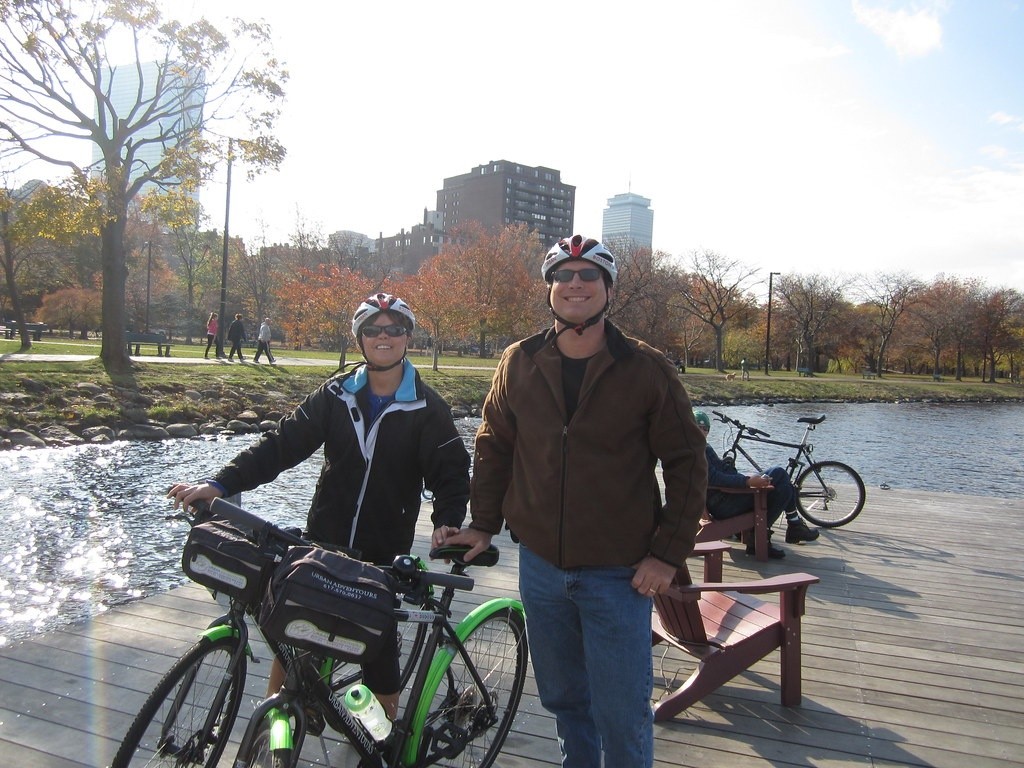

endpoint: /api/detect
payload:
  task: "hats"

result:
[693,411,710,427]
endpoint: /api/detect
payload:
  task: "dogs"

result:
[726,372,737,382]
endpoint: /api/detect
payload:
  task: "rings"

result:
[445,526,449,531]
[649,588,656,593]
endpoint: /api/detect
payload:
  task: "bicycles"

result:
[712,410,866,529]
[111,487,530,768]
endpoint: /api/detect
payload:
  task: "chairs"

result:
[695,485,775,563]
[649,538,821,723]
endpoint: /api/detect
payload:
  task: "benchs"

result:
[797,367,812,376]
[932,375,945,383]
[861,370,876,380]
[127,332,175,357]
[6,320,49,342]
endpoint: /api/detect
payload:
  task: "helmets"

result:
[540,235,618,288]
[351,291,416,340]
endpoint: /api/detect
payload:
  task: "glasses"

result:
[362,325,408,338]
[550,269,606,283]
[698,425,709,431]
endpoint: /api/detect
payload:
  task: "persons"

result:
[167,292,471,721]
[204,312,222,359]
[227,314,247,362]
[446,234,708,768]
[252,318,276,364]
[741,357,750,381]
[693,411,819,558]
[681,358,686,373]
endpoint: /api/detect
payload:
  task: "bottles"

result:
[345,684,392,741]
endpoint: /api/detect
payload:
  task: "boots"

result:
[746,528,785,559]
[785,519,819,543]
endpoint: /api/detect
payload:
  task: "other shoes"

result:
[254,360,259,364]
[240,358,245,361]
[270,360,276,364]
[228,358,234,362]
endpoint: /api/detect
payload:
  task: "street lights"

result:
[765,272,781,375]
[216,138,246,358]
[143,241,152,333]
